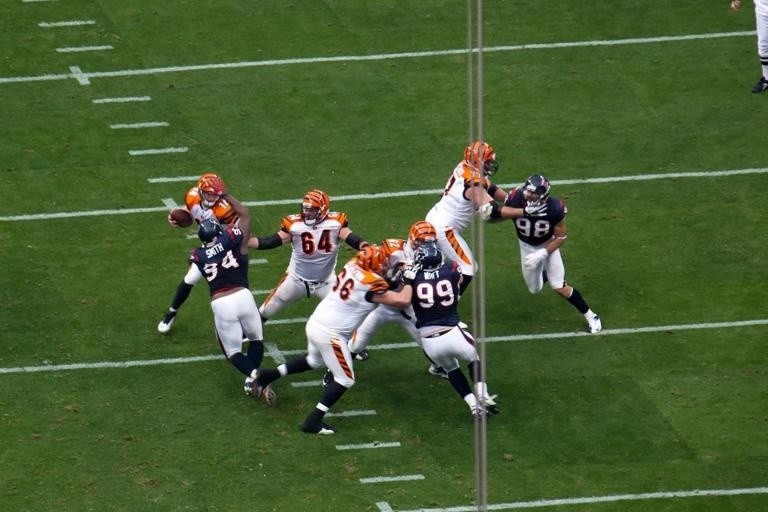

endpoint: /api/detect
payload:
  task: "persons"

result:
[506,173,601,334]
[426,143,548,329]
[158,173,239,333]
[409,245,499,420]
[321,221,449,388]
[244,242,419,435]
[189,191,277,406]
[732,1,768,94]
[242,189,370,361]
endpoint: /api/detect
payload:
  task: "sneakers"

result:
[157,306,177,334]
[322,370,335,388]
[427,364,450,380]
[300,420,338,436]
[355,350,369,361]
[478,397,503,415]
[587,313,602,335]
[244,368,277,407]
[470,405,490,420]
[752,76,768,93]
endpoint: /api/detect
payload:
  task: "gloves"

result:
[369,278,394,295]
[402,262,421,283]
[522,203,549,218]
[206,175,229,199]
[523,247,549,272]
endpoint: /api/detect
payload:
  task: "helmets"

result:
[524,175,550,203]
[197,217,221,243]
[302,189,330,226]
[409,220,437,250]
[463,140,496,173]
[356,244,386,274]
[413,244,443,272]
[197,173,226,196]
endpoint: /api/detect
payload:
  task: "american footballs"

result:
[170,210,192,228]
